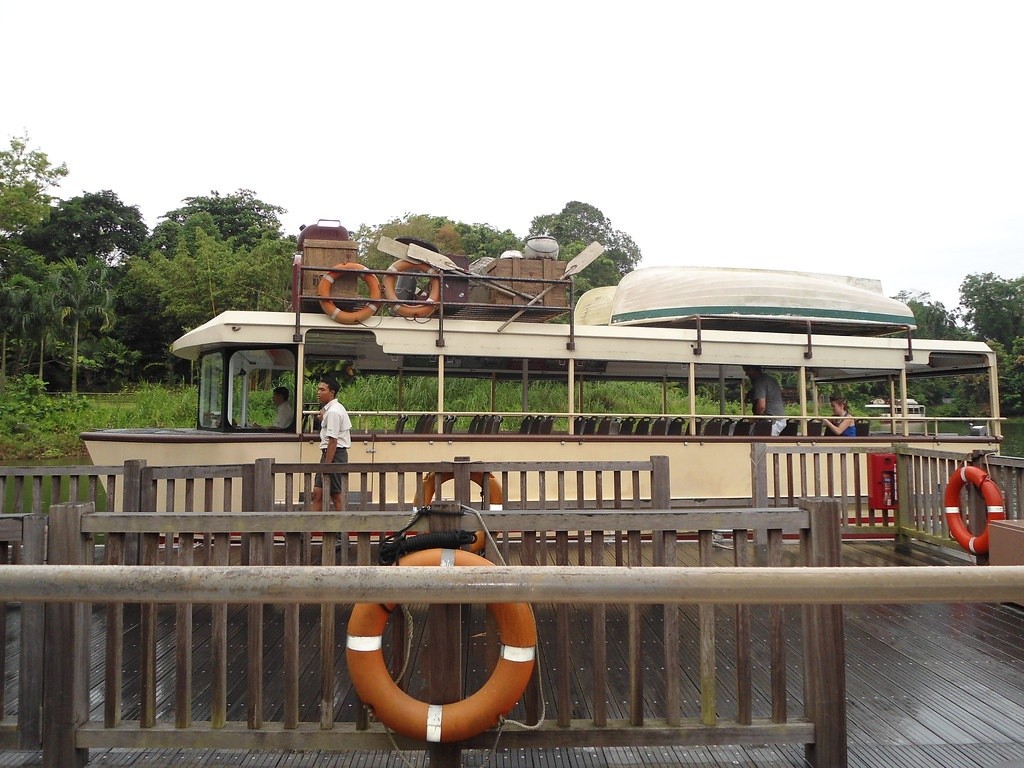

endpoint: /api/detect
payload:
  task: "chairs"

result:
[393,411,872,441]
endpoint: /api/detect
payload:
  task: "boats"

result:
[76,217,1007,541]
[860,398,927,422]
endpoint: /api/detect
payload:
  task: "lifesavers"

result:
[382,259,440,318]
[412,472,503,551]
[345,548,537,742]
[944,466,1007,554]
[318,262,382,325]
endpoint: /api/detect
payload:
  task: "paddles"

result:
[377,235,605,332]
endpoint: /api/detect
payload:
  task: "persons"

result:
[741,366,787,436]
[809,396,856,437]
[296,376,353,546]
[252,386,293,430]
